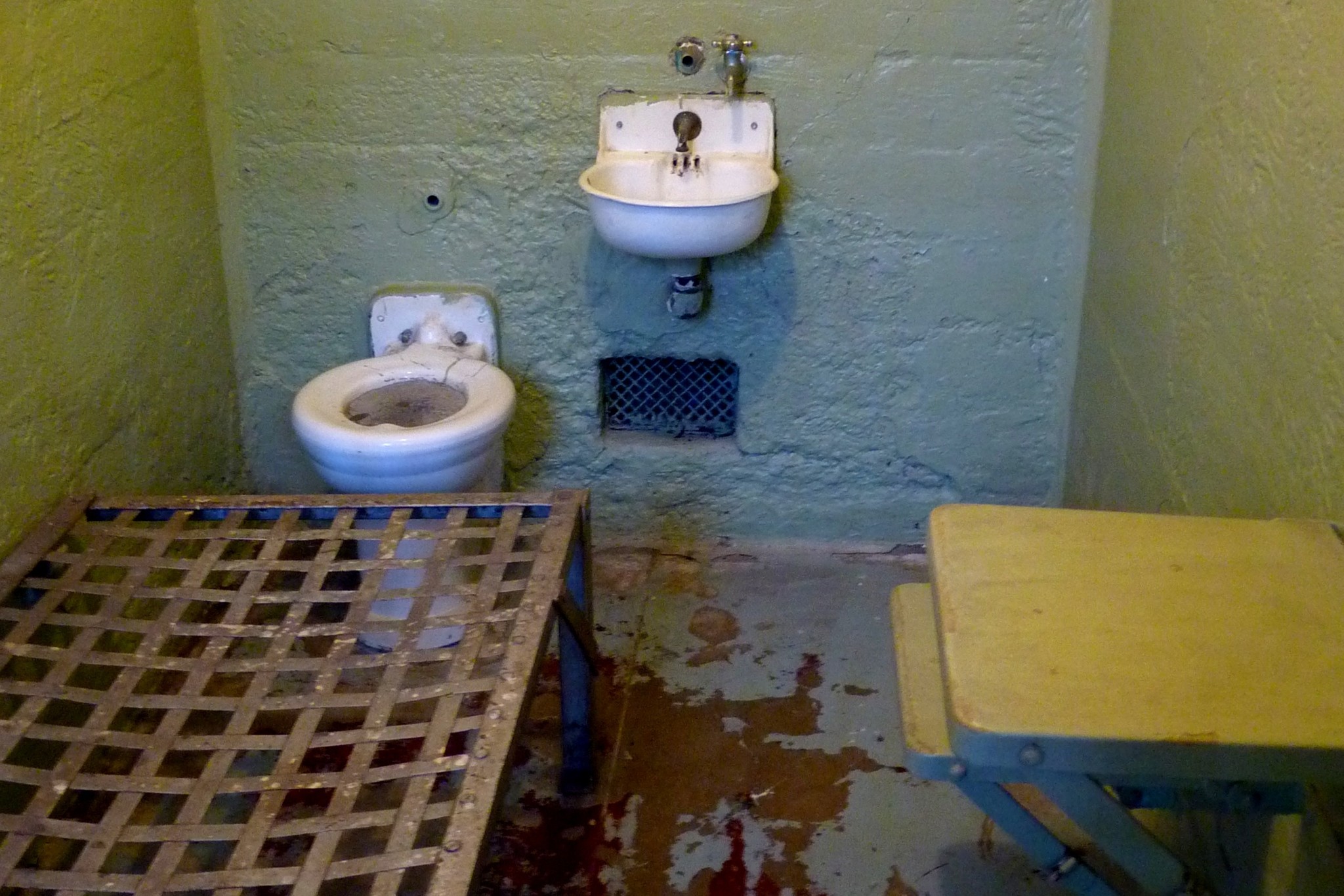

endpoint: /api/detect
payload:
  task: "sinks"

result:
[577,147,780,260]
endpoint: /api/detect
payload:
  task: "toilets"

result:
[290,283,517,654]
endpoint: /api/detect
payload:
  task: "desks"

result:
[893,504,1344,896]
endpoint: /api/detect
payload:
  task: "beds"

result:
[1,482,599,895]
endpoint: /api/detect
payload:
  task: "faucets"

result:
[676,112,695,153]
[711,33,753,103]
